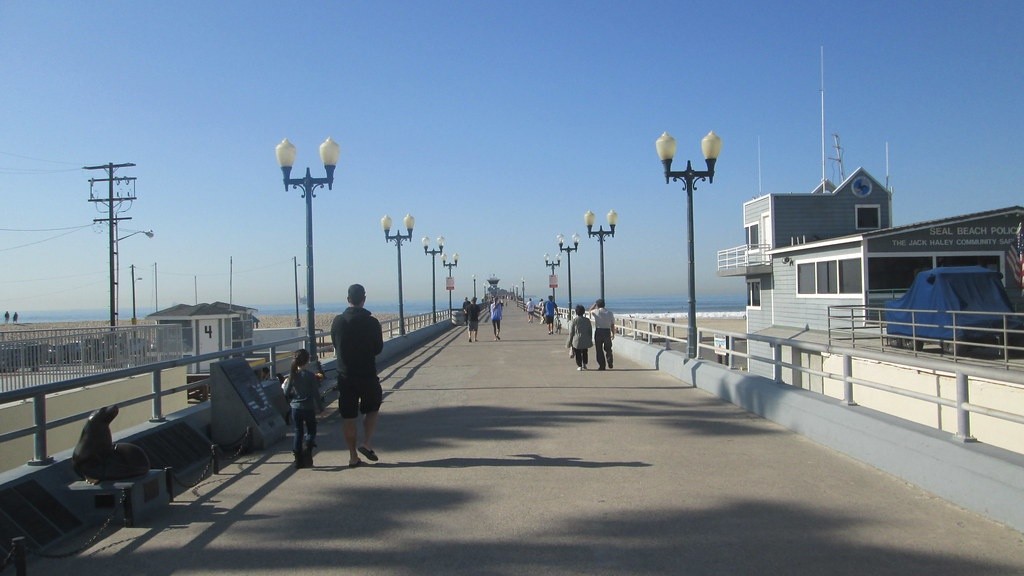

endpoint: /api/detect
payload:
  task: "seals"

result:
[71,405,151,485]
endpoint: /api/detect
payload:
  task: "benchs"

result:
[274,357,340,426]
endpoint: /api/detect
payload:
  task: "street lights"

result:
[555,233,581,322]
[109,229,154,327]
[543,252,561,303]
[275,136,340,362]
[521,276,525,308]
[653,130,722,359]
[132,278,143,318]
[584,209,619,300]
[441,252,459,317]
[295,264,302,321]
[422,235,445,324]
[381,213,414,335]
[472,273,477,298]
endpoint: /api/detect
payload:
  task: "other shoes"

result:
[474,340,478,342]
[468,337,471,342]
[551,330,553,334]
[294,452,303,469]
[494,337,497,341]
[303,445,313,467]
[549,331,551,334]
[598,367,605,370]
[496,334,500,340]
[583,365,587,369]
[609,364,613,368]
[577,367,582,371]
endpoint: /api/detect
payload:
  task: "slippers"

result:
[349,457,361,467]
[356,443,379,465]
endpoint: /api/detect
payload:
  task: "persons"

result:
[542,295,558,334]
[589,299,615,370]
[566,305,593,371]
[284,349,323,468]
[526,298,534,323]
[4,311,10,323]
[538,298,545,323]
[467,297,480,342]
[331,284,384,467]
[490,296,502,341]
[463,296,471,326]
[13,312,18,323]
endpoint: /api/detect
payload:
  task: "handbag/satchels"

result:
[540,317,545,325]
[569,347,575,358]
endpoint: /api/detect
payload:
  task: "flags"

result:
[1005,228,1024,289]
